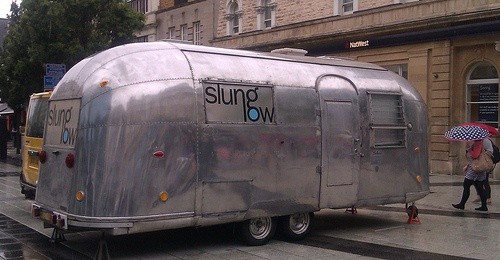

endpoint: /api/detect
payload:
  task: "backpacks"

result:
[487,138,500,164]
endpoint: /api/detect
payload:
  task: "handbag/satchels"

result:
[471,141,495,172]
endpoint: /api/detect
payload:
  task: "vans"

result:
[20,91,52,199]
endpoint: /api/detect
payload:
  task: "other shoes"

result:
[452,203,465,210]
[475,207,488,211]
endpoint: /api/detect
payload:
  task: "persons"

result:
[12,120,20,155]
[17,120,26,151]
[451,139,488,211]
[469,137,495,203]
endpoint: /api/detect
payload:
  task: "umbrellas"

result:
[439,119,497,153]
